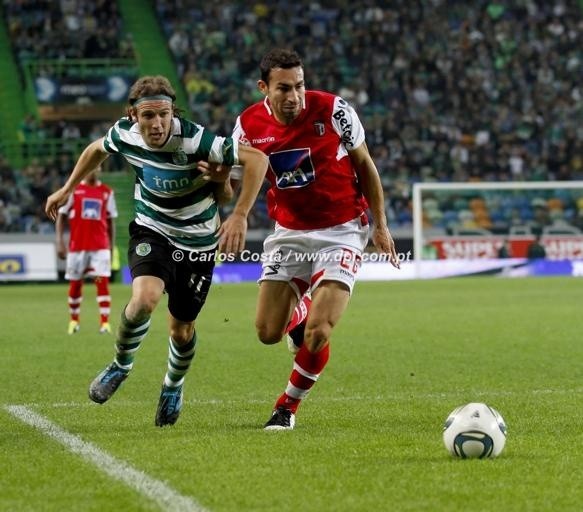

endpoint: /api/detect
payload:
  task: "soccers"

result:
[443,402,507,458]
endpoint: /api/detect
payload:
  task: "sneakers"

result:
[262,406,296,432]
[154,379,184,429]
[68,320,80,335]
[99,321,112,335]
[88,361,130,405]
[286,319,307,356]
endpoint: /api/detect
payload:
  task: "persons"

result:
[1,1,582,259]
[196,45,402,432]
[54,164,119,337]
[44,74,269,428]
